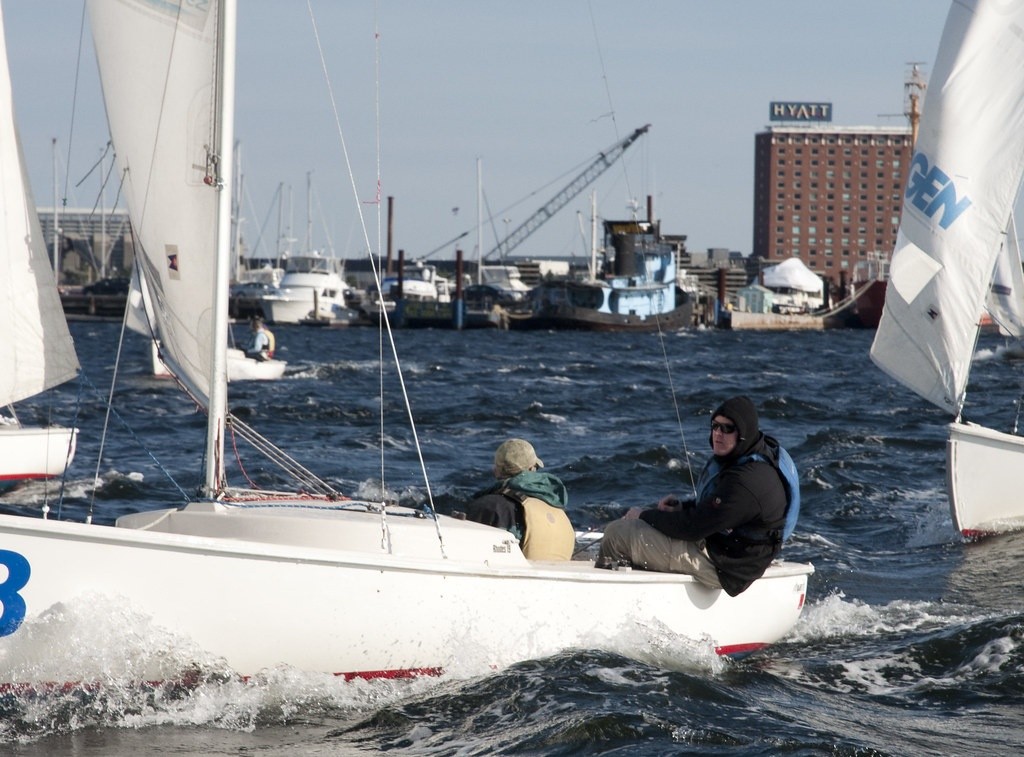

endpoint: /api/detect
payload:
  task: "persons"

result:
[243,316,275,362]
[595,394,801,597]
[464,439,576,565]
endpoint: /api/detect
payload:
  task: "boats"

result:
[151,336,293,382]
[43,136,1008,324]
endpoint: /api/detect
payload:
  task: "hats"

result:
[493,439,544,479]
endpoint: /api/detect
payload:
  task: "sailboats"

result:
[2,0,816,699]
[868,2,1023,540]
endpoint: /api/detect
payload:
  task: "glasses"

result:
[710,419,738,434]
